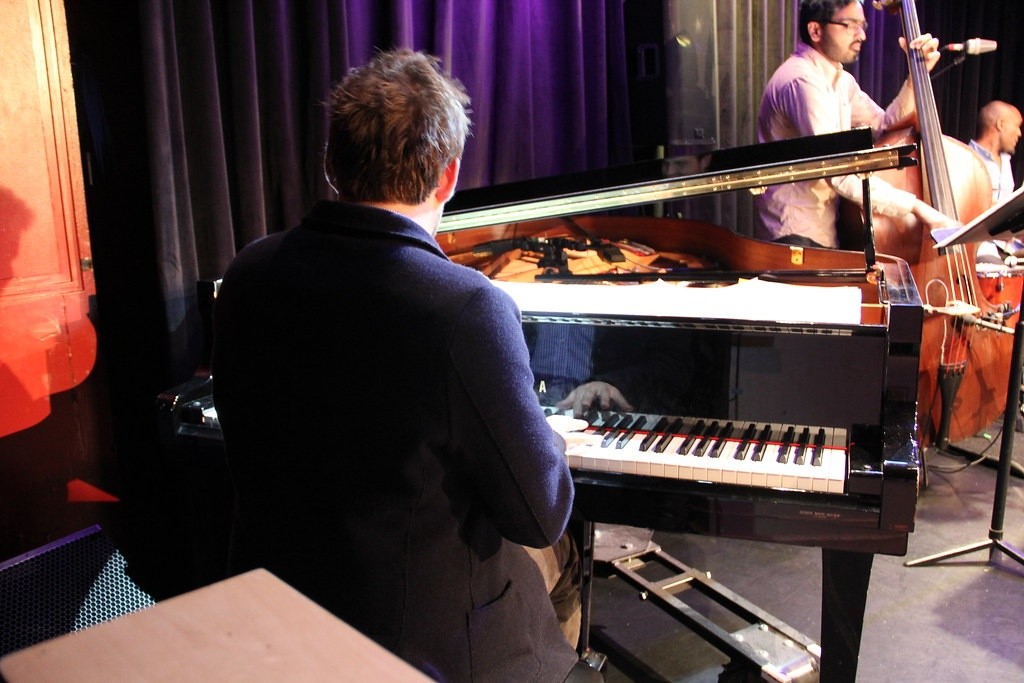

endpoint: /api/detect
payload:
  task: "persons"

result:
[202,46,606,683]
[750,0,967,252]
[521,321,730,418]
[964,99,1024,208]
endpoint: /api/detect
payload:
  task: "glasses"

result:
[829,21,868,32]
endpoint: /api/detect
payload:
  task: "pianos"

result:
[168,126,928,683]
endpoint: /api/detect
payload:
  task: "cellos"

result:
[828,0,1024,485]
